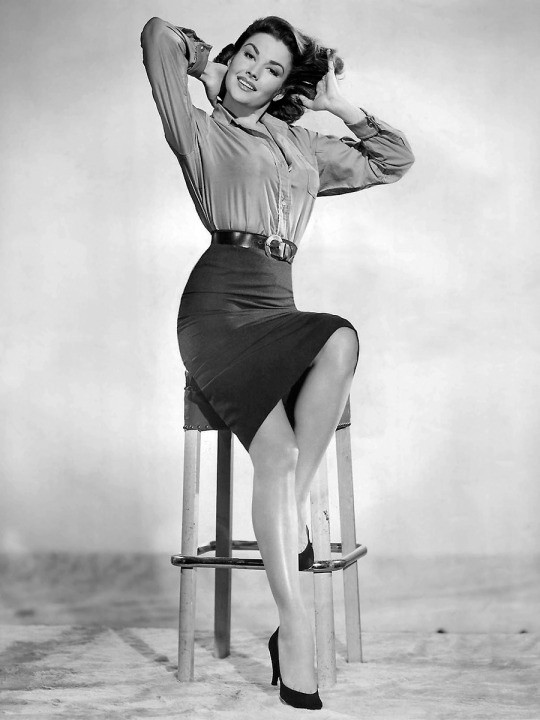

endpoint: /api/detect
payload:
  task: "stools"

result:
[172,369,366,685]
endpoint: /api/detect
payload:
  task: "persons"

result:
[139,16,415,710]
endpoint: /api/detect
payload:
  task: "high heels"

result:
[298,526,314,570]
[268,626,323,710]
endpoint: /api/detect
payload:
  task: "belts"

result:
[211,228,298,264]
